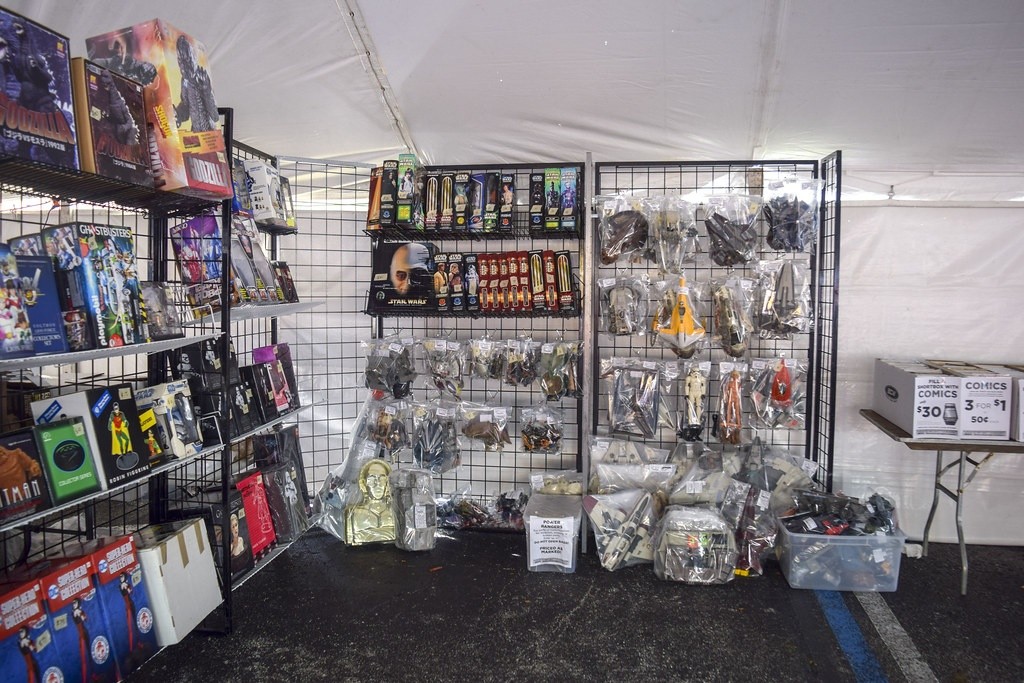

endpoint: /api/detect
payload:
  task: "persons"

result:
[382,168,577,295]
[267,359,292,409]
[345,460,400,547]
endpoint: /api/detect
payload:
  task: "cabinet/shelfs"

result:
[168,128,328,594]
[0,106,234,683]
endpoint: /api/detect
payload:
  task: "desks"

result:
[859,409,1024,596]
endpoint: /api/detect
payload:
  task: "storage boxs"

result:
[775,516,909,592]
[871,357,1024,442]
[522,494,582,573]
[360,152,581,316]
[0,8,312,683]
